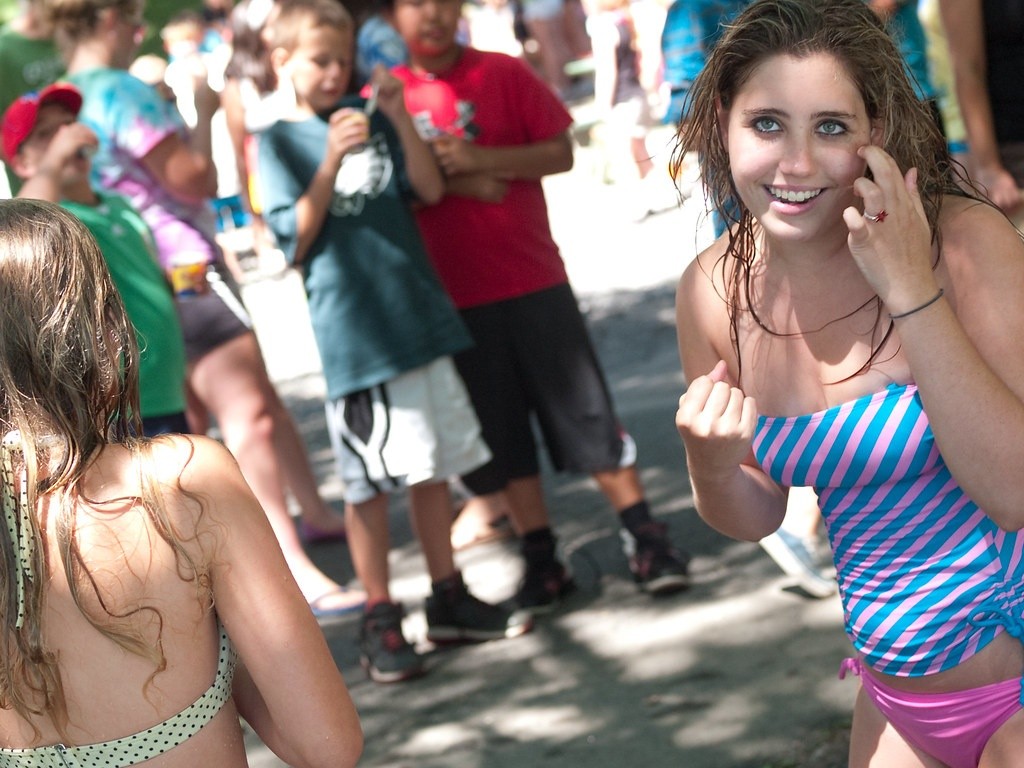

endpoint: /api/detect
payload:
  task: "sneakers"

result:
[518,535,575,613]
[355,600,423,682]
[423,574,534,640]
[617,519,690,594]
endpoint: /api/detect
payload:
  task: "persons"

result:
[0,196,365,768]
[669,0,1024,768]
[0,0,1024,682]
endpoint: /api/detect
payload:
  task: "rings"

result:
[863,209,890,224]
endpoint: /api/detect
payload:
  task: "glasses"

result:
[114,9,147,48]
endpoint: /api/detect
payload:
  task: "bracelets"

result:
[887,286,945,323]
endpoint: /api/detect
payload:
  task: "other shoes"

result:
[302,518,347,550]
[295,580,367,619]
[758,529,840,598]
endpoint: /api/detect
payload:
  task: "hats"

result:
[1,82,84,164]
